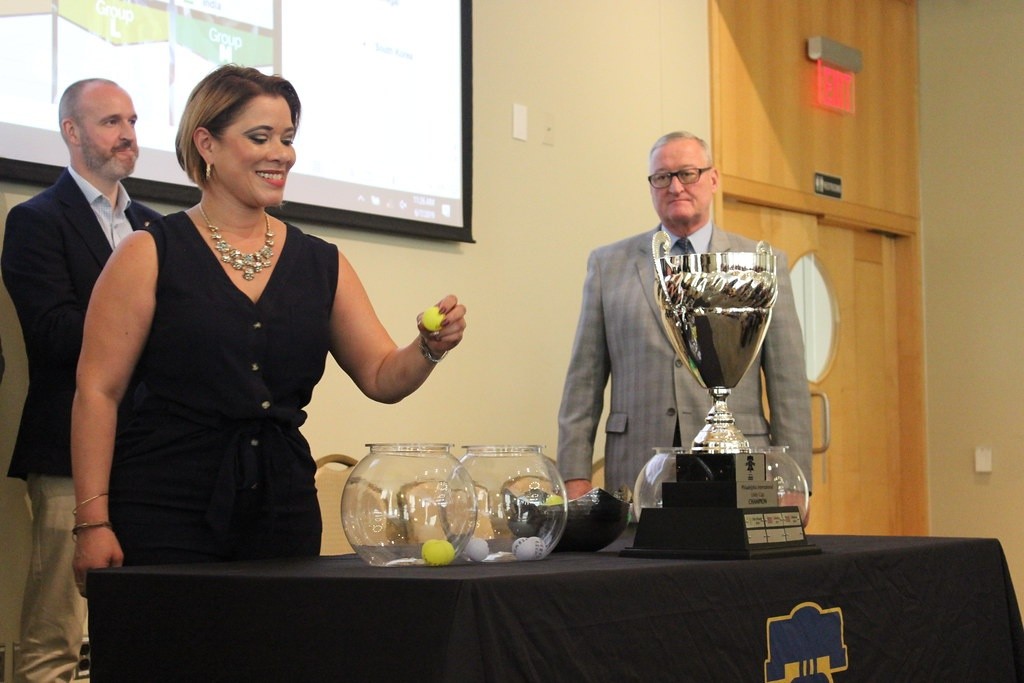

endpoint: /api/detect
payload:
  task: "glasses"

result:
[647,166,712,189]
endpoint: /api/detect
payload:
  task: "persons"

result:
[1,78,164,683]
[555,130,813,528]
[69,64,467,598]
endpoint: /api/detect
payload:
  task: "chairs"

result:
[315,454,603,559]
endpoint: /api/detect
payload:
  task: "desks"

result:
[83,534,1024,683]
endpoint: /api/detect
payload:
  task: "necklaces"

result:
[199,203,276,281]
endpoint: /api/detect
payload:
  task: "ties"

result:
[676,237,692,253]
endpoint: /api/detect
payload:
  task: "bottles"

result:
[341,442,476,568]
[632,446,808,524]
[439,444,568,563]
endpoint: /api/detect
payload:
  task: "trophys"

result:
[619,230,822,561]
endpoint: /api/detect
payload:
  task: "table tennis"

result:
[511,536,547,560]
[546,494,563,505]
[423,306,447,331]
[420,538,456,565]
[466,538,490,562]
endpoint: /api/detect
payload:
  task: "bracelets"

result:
[419,338,448,364]
[71,492,112,543]
